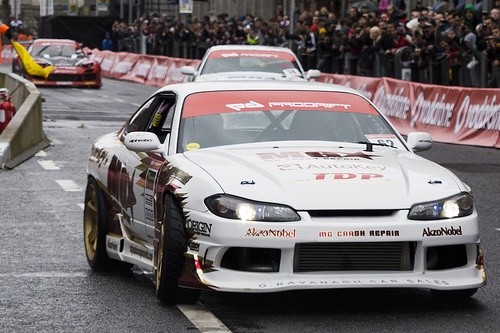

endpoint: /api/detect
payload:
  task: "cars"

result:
[82,80,487,311]
[12,39,102,90]
[181,45,310,83]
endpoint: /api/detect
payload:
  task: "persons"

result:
[8,0,500,90]
